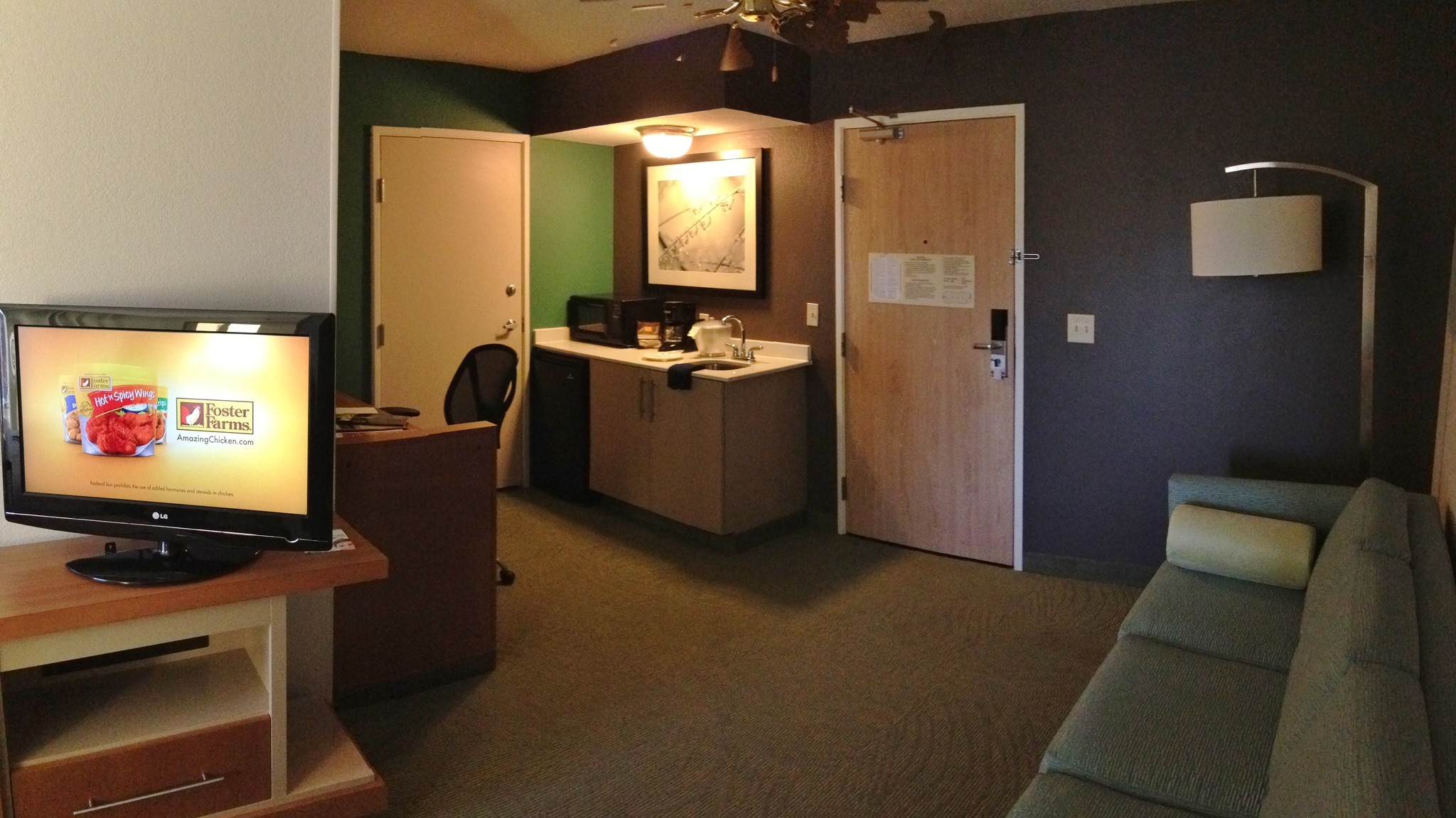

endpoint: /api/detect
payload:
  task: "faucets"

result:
[721,315,746,359]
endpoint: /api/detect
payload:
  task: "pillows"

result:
[1300,481,1412,638]
[1268,551,1422,790]
[1258,664,1441,818]
[1166,505,1317,591]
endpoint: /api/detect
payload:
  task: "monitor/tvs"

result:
[1,303,335,551]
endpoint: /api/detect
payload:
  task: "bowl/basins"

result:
[687,316,732,357]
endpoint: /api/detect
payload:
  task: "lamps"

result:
[1190,161,1380,488]
[578,0,847,82]
[635,125,698,159]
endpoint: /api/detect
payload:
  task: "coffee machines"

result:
[658,300,699,354]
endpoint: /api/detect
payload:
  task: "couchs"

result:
[1005,472,1456,818]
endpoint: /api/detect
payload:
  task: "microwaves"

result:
[567,293,663,349]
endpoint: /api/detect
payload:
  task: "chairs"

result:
[379,343,517,586]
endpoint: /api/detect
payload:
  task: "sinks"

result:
[688,360,751,372]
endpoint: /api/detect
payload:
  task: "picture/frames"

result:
[641,146,765,300]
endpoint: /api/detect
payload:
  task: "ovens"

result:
[527,345,591,509]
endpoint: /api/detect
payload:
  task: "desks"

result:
[334,389,498,705]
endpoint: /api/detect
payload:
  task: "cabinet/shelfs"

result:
[530,347,595,507]
[1,512,390,818]
[587,358,808,534]
